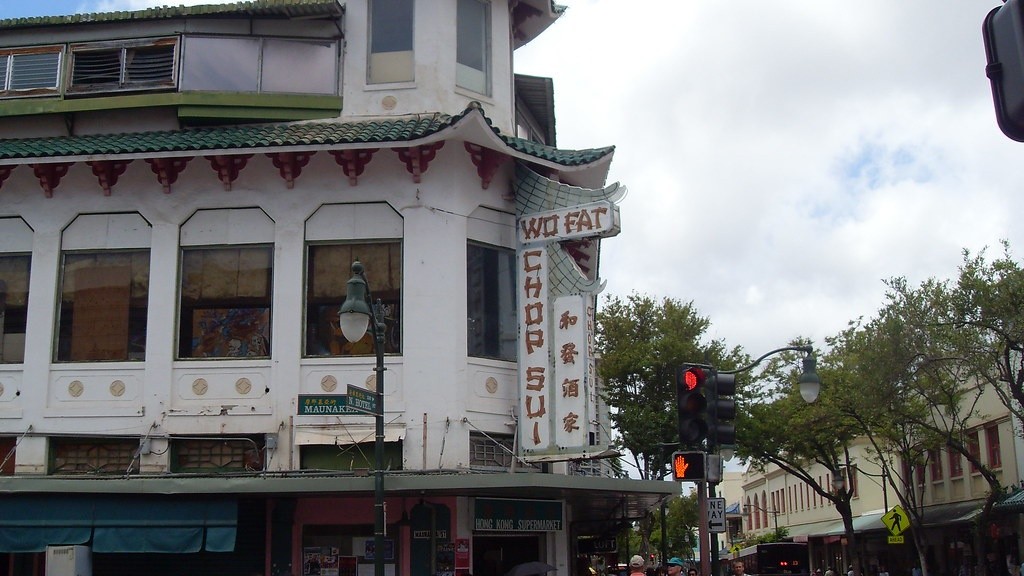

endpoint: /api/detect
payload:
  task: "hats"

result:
[665,557,684,567]
[828,566,831,568]
[630,555,645,567]
[816,568,821,572]
[849,565,853,569]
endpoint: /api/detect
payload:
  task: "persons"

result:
[596,555,1024,576]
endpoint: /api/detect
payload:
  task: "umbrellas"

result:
[505,562,559,576]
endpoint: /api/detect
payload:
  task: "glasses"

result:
[667,563,679,567]
[689,574,695,575]
[735,566,741,568]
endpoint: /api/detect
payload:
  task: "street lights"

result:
[743,505,780,542]
[336,254,389,575]
[708,343,821,576]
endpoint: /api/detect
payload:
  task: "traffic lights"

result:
[676,366,708,445]
[673,451,706,482]
[714,370,737,445]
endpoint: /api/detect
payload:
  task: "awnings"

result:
[777,497,989,542]
[0,494,239,554]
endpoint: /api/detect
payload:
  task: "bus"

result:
[725,542,811,576]
